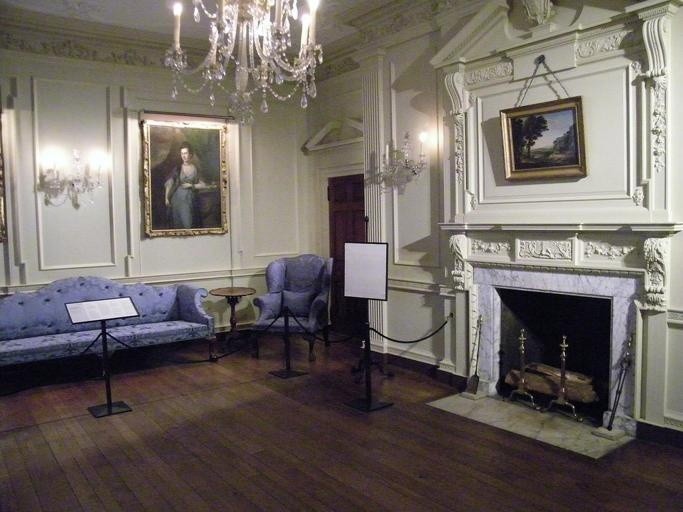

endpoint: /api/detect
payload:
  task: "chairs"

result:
[252,253,334,371]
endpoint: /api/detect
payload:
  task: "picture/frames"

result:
[498,95,587,179]
[139,110,235,240]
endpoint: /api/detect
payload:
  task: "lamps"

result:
[378,137,427,183]
[163,1,326,117]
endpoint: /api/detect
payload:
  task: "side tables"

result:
[209,288,256,362]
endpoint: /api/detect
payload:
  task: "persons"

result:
[165,141,208,228]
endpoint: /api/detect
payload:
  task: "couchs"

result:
[0,274,219,366]
